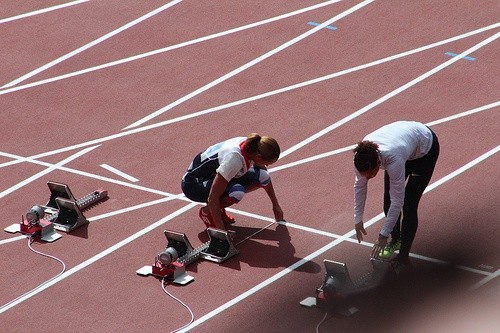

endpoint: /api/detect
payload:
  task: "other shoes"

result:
[379,238,401,258]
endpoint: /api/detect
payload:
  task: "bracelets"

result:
[378,233,390,239]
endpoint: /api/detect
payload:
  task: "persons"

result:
[181,132,284,230]
[353,121,441,262]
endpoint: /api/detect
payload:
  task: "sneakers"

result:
[198,207,215,229]
[221,209,234,223]
